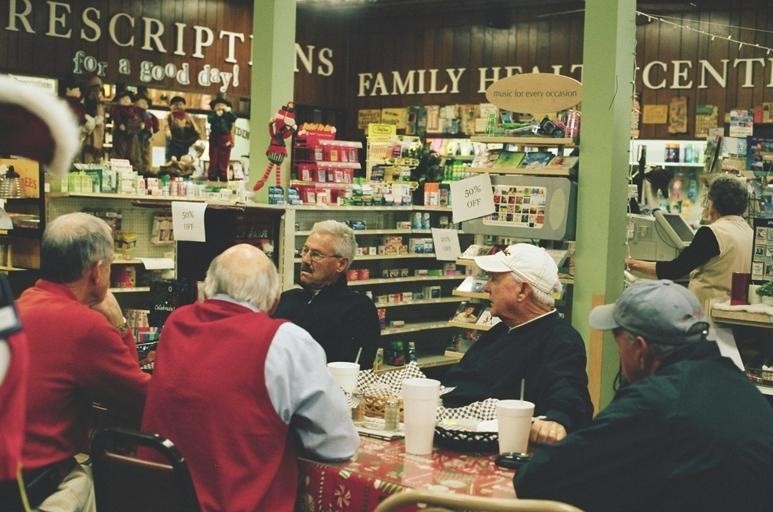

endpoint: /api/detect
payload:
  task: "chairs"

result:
[87,425,200,512]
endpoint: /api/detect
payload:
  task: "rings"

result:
[547,429,559,436]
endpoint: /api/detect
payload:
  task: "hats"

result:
[589,279,710,346]
[474,242,559,294]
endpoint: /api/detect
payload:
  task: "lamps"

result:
[0,165,26,200]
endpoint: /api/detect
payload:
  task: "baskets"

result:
[434,425,499,452]
[362,394,403,421]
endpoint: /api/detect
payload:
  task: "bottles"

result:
[383,337,418,367]
[662,142,708,163]
[382,398,400,431]
[161,173,207,200]
[423,161,470,208]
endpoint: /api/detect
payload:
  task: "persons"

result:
[134,240,358,511]
[15,212,155,510]
[621,174,754,377]
[0,271,34,511]
[500,273,770,511]
[58,73,302,197]
[434,243,591,449]
[265,216,380,371]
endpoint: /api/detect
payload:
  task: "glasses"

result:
[298,246,342,263]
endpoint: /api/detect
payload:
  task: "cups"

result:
[326,361,359,394]
[730,272,750,305]
[494,399,536,459]
[401,378,440,455]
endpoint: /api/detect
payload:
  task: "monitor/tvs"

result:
[654,211,697,250]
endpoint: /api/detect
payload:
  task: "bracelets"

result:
[112,315,129,333]
[624,257,632,273]
